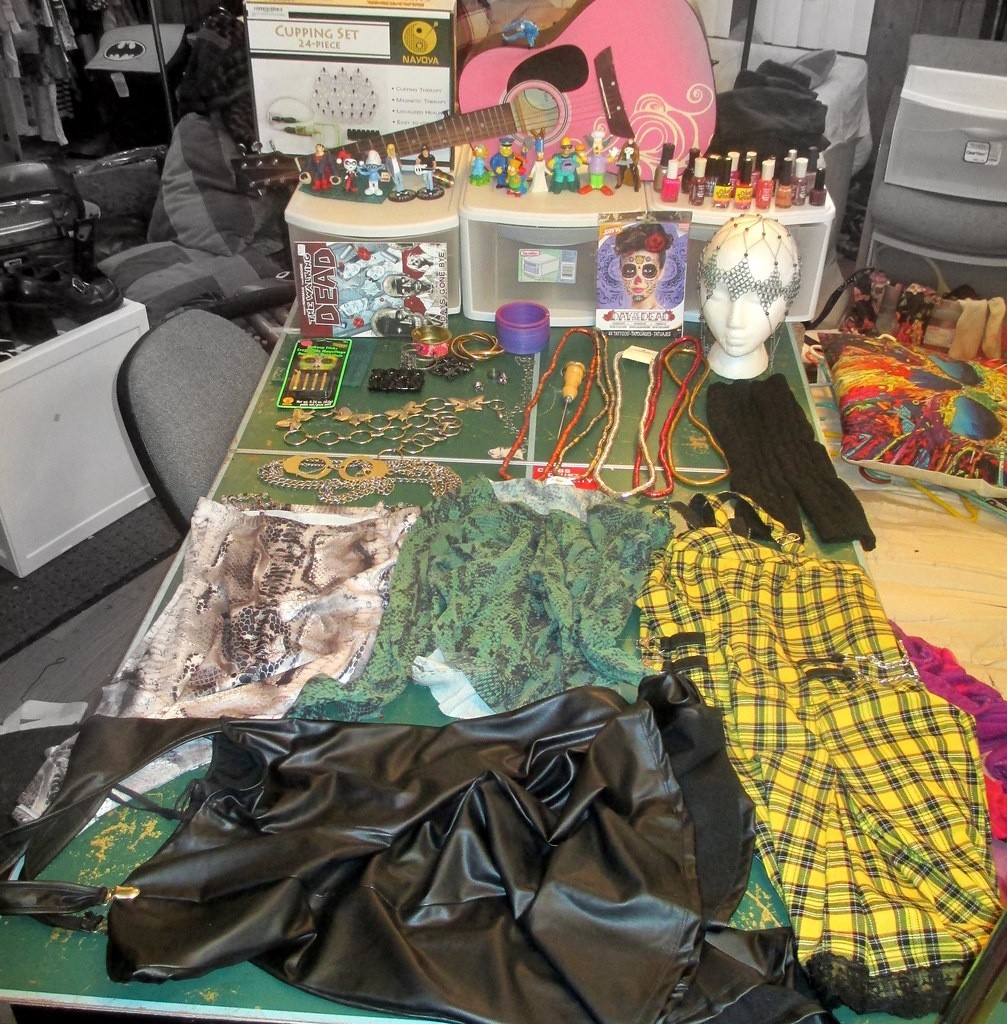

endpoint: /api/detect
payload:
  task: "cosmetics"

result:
[653,143,827,210]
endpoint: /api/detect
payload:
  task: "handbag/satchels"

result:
[702,60,831,179]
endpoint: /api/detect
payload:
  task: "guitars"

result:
[228,1,718,201]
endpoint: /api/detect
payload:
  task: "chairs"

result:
[117,308,270,533]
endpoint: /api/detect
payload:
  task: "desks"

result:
[0,325,862,1023]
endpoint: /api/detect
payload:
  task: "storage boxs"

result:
[285,147,461,313]
[646,177,835,323]
[1,300,148,580]
[463,147,643,326]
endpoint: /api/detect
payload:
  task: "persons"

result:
[303,143,436,196]
[337,243,447,337]
[698,212,802,380]
[469,129,642,198]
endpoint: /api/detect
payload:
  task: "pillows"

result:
[94,241,291,313]
[817,331,1007,497]
[147,108,269,262]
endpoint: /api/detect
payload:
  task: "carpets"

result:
[1,498,185,664]
